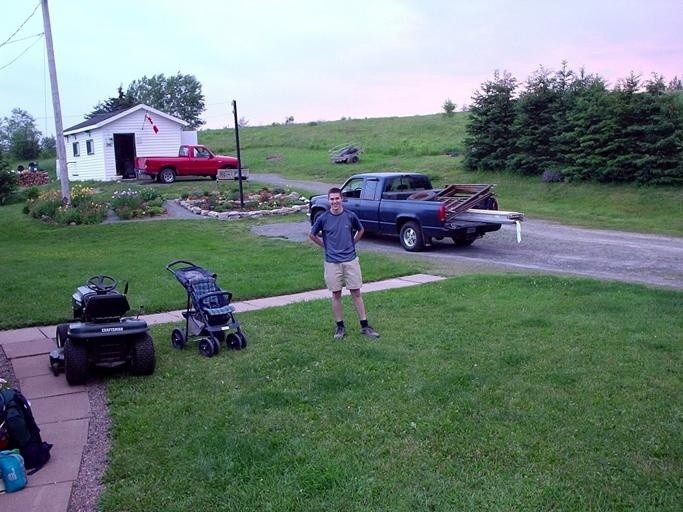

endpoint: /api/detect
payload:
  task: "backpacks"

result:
[0,386,51,471]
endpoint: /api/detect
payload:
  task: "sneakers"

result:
[359,327,380,338]
[333,326,346,340]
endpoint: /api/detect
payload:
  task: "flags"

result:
[146,115,158,135]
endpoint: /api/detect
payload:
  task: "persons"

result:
[310,187,380,339]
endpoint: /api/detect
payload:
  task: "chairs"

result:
[80,293,131,324]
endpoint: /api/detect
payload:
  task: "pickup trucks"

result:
[309,172,501,253]
[135,144,238,183]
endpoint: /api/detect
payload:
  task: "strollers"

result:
[166,259,246,357]
